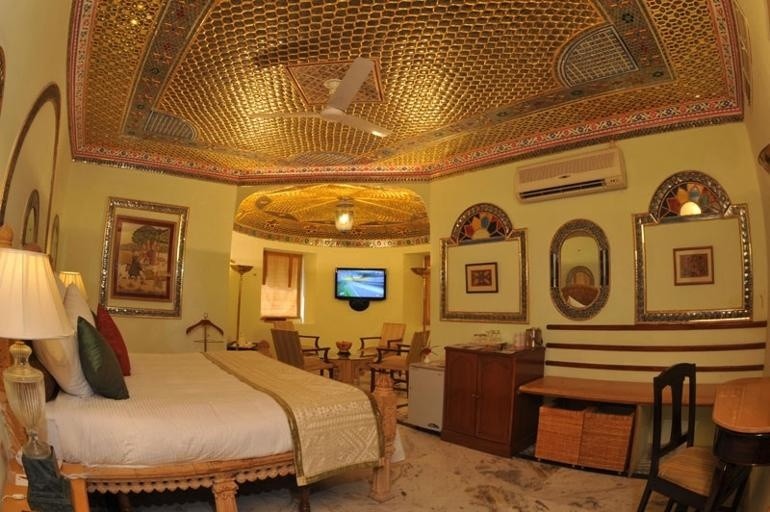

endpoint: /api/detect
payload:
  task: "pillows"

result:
[64,280,97,329]
[98,301,132,377]
[76,316,130,398]
[30,333,95,398]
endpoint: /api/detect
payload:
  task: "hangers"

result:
[185,313,224,336]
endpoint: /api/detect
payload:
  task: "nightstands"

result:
[0,478,91,512]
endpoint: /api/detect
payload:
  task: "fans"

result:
[243,57,395,139]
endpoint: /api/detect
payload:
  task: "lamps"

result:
[333,196,356,236]
[57,269,87,299]
[228,264,253,351]
[0,245,82,474]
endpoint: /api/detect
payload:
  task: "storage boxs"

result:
[579,404,636,476]
[560,441,563,442]
[534,401,590,468]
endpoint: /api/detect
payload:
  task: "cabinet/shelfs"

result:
[407,360,446,436]
[444,341,547,460]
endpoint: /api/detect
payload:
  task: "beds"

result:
[0,225,406,503]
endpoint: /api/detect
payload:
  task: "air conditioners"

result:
[514,147,629,203]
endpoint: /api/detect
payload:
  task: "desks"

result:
[317,355,375,388]
[714,375,770,512]
[520,375,716,478]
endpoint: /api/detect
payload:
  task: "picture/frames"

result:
[98,197,191,319]
[631,169,755,330]
[550,217,610,322]
[439,200,529,325]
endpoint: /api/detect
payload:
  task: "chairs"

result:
[269,329,339,381]
[358,322,408,378]
[638,361,749,511]
[368,329,431,399]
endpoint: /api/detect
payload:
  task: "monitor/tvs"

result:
[334,266,387,300]
